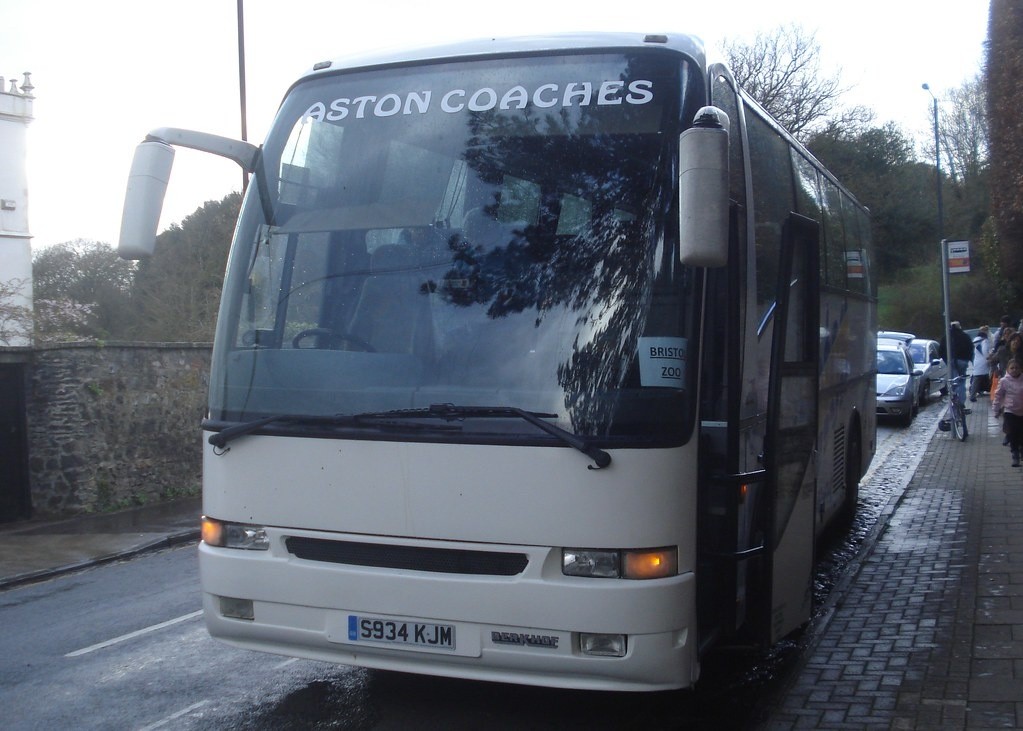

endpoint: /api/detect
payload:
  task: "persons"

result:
[396,206,533,350]
[939,321,974,413]
[969,315,1023,466]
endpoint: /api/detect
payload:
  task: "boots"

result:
[1010,451,1020,466]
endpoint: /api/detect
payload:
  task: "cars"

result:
[877,330,947,404]
[877,338,924,429]
[963,326,1000,349]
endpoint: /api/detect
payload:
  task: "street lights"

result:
[921,83,960,441]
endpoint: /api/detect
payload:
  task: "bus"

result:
[117,30,877,702]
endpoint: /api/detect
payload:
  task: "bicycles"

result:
[934,375,974,441]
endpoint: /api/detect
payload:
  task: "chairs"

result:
[341,245,434,358]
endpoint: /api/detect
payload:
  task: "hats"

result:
[999,314,1009,323]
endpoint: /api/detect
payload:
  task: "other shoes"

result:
[970,397,977,402]
[1003,435,1009,445]
